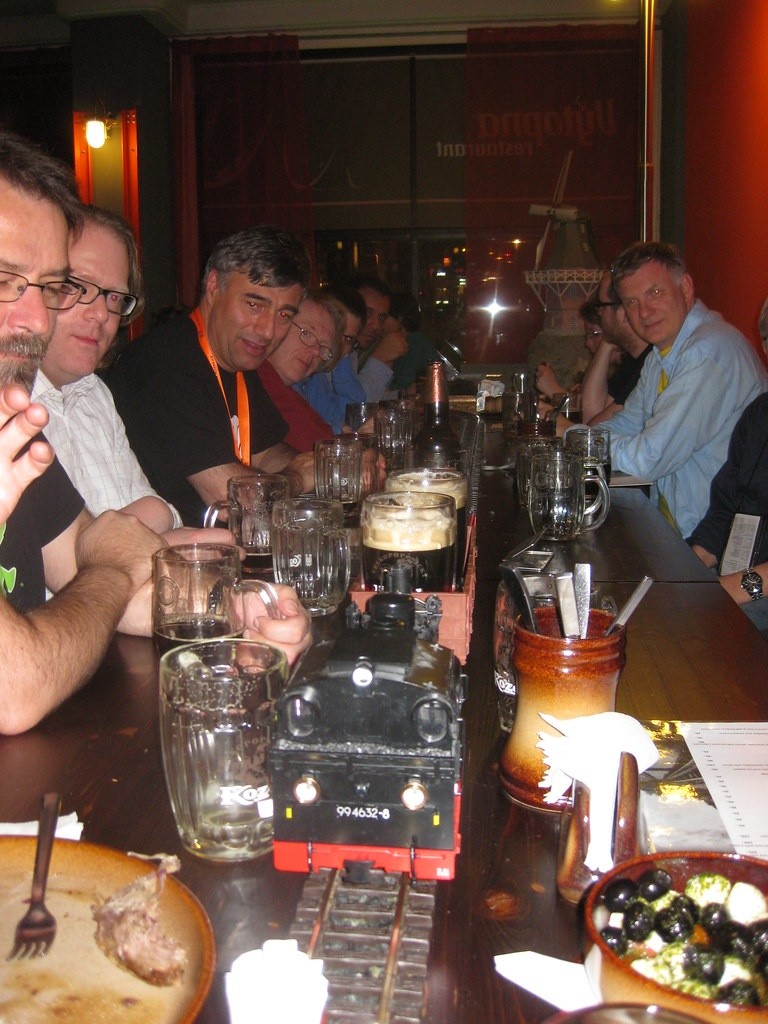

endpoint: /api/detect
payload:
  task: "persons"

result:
[687,296,768,632]
[256,288,345,453]
[534,261,653,428]
[357,291,438,390]
[292,284,366,435]
[0,130,313,736]
[26,203,248,564]
[538,240,768,540]
[347,272,415,405]
[106,221,386,522]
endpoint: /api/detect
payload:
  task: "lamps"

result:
[83,110,110,149]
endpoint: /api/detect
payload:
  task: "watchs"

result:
[741,567,763,600]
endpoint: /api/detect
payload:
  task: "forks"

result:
[7,792,63,959]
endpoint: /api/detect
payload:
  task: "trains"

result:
[266,460,480,886]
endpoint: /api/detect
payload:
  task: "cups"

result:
[204,474,288,574]
[385,468,468,582]
[360,491,456,592]
[158,638,290,862]
[272,498,351,617]
[515,429,612,541]
[315,439,362,517]
[345,400,418,470]
[499,608,626,814]
[493,573,618,695]
[151,542,245,657]
[333,432,378,508]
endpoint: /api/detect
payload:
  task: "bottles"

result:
[414,360,463,470]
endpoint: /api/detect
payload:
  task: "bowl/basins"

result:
[585,851,768,1024]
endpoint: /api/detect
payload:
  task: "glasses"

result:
[292,321,335,362]
[67,276,138,317]
[605,248,683,275]
[0,271,87,310]
[590,301,623,313]
[341,334,358,346]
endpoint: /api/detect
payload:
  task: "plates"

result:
[0,834,216,1024]
